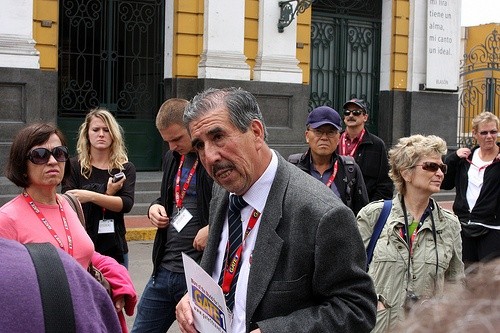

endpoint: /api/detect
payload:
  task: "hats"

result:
[343,98,367,114]
[307,106,342,130]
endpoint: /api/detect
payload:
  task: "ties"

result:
[218,195,249,313]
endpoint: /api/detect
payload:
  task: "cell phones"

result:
[112,172,124,183]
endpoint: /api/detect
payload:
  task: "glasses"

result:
[308,129,338,138]
[413,162,447,174]
[477,130,498,135]
[343,109,363,116]
[27,145,71,165]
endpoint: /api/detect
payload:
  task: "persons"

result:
[0,109,136,333]
[131,87,394,333]
[356,112,500,333]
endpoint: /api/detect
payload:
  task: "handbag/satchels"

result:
[88,265,110,297]
